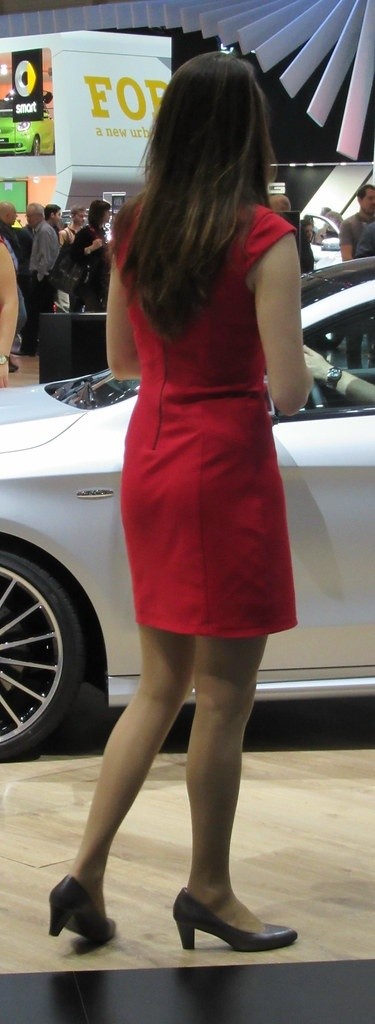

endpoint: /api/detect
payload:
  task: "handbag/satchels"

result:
[50,230,89,295]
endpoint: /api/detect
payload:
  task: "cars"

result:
[1,254,375,762]
[303,212,342,271]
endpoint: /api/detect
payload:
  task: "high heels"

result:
[48,874,115,941]
[174,889,297,953]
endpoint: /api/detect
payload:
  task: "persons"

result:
[301,344,375,405]
[269,183,375,276]
[46,51,312,951]
[0,200,111,388]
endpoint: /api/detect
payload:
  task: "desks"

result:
[38,312,109,384]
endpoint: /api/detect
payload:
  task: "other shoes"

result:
[8,360,18,372]
[11,349,35,357]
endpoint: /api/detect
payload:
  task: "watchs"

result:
[0,354,10,365]
[327,367,341,389]
